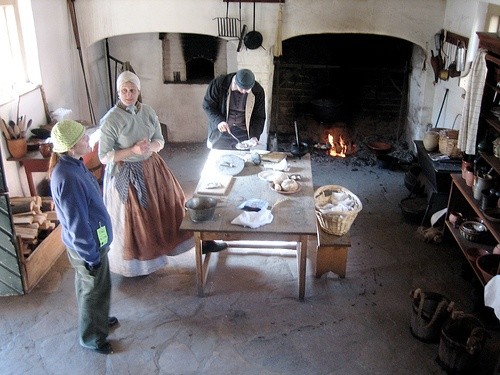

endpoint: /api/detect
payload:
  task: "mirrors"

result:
[160,33,227,84]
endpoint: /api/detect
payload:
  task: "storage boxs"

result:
[313,184,363,236]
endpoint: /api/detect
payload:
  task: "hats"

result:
[52,120,86,153]
[117,71,141,92]
[235,69,257,89]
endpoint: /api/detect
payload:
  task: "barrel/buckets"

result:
[185,197,218,222]
[240,199,269,224]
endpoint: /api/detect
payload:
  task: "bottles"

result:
[449,154,500,283]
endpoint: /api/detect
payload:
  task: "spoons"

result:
[230,131,241,143]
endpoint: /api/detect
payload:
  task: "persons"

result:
[48,120,120,355]
[96,71,229,278]
[201,69,266,152]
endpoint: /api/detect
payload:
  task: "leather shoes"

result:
[95,342,115,355]
[107,317,118,326]
[201,241,228,254]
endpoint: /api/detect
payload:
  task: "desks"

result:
[6,123,108,197]
[178,148,317,298]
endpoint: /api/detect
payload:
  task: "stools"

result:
[315,220,351,279]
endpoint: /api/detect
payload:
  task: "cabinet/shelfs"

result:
[433,28,500,326]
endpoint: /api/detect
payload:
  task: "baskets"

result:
[439,112,465,160]
[314,184,361,235]
[400,196,429,222]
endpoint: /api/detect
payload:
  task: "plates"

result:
[269,180,303,195]
[257,170,282,182]
[235,140,257,150]
